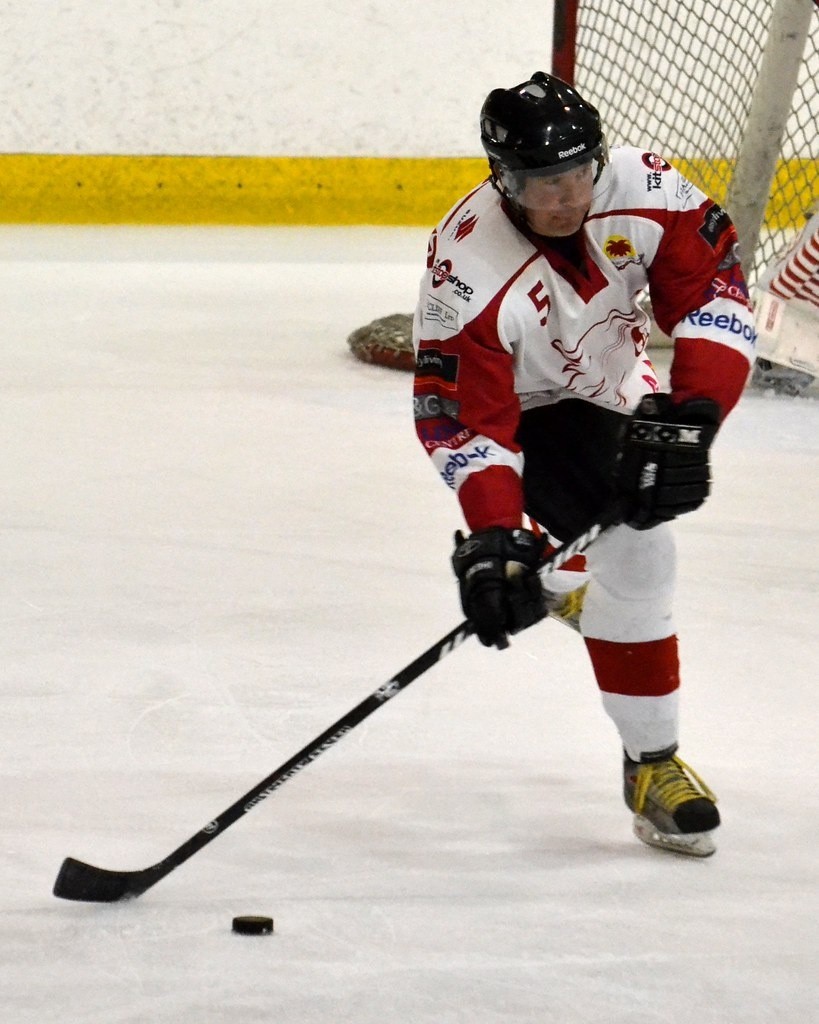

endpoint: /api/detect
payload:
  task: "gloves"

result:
[607,392,722,530]
[453,525,548,651]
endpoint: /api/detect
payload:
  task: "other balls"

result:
[230,914,275,936]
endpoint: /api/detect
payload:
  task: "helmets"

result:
[479,71,604,190]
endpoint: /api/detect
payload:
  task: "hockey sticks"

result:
[50,508,623,906]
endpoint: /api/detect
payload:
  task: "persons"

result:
[412,71,759,836]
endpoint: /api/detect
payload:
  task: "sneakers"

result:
[550,578,587,632]
[624,746,720,857]
